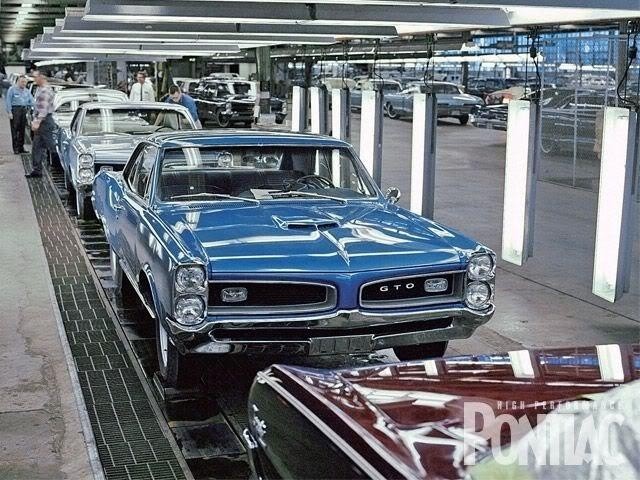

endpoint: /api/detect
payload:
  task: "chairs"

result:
[134,169,309,203]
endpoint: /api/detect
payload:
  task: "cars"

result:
[90,129,498,388]
[384,82,484,125]
[470,88,636,155]
[10,72,202,219]
[309,78,357,109]
[243,339,639,479]
[173,73,287,127]
[349,79,403,113]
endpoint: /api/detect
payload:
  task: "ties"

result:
[141,85,142,101]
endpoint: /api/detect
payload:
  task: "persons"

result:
[153,85,203,131]
[6,74,35,154]
[0,71,7,97]
[128,71,157,125]
[24,67,63,178]
[27,63,85,85]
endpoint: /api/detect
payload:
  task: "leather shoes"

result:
[15,150,29,154]
[25,173,42,178]
